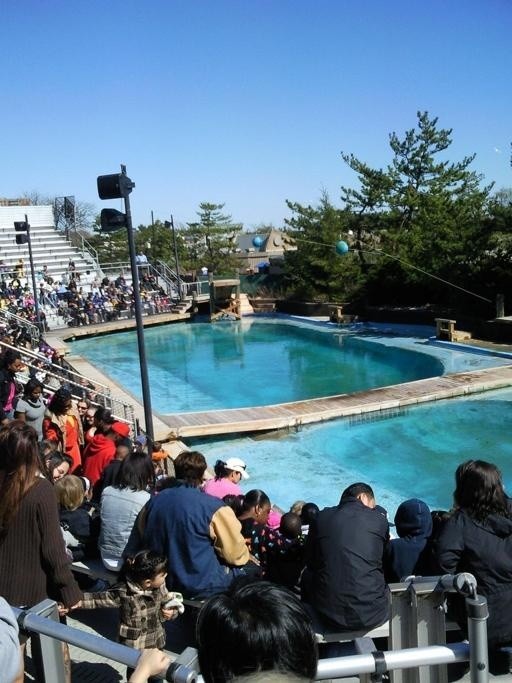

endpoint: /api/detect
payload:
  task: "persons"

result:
[0,257,171,517]
[123,451,261,605]
[58,550,185,683]
[235,489,281,562]
[204,457,250,500]
[301,503,319,525]
[54,474,105,591]
[1,420,83,682]
[301,482,393,658]
[262,513,307,588]
[129,648,168,682]
[197,575,319,683]
[382,498,440,580]
[435,460,511,674]
[99,452,157,571]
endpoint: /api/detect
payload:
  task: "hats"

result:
[112,421,130,437]
[225,458,250,480]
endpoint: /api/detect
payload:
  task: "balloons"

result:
[336,239,350,255]
[252,236,263,248]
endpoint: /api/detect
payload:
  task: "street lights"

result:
[97,163,156,462]
[165,213,182,301]
[14,213,43,338]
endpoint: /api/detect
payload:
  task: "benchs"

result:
[69,556,463,646]
[0,226,177,317]
